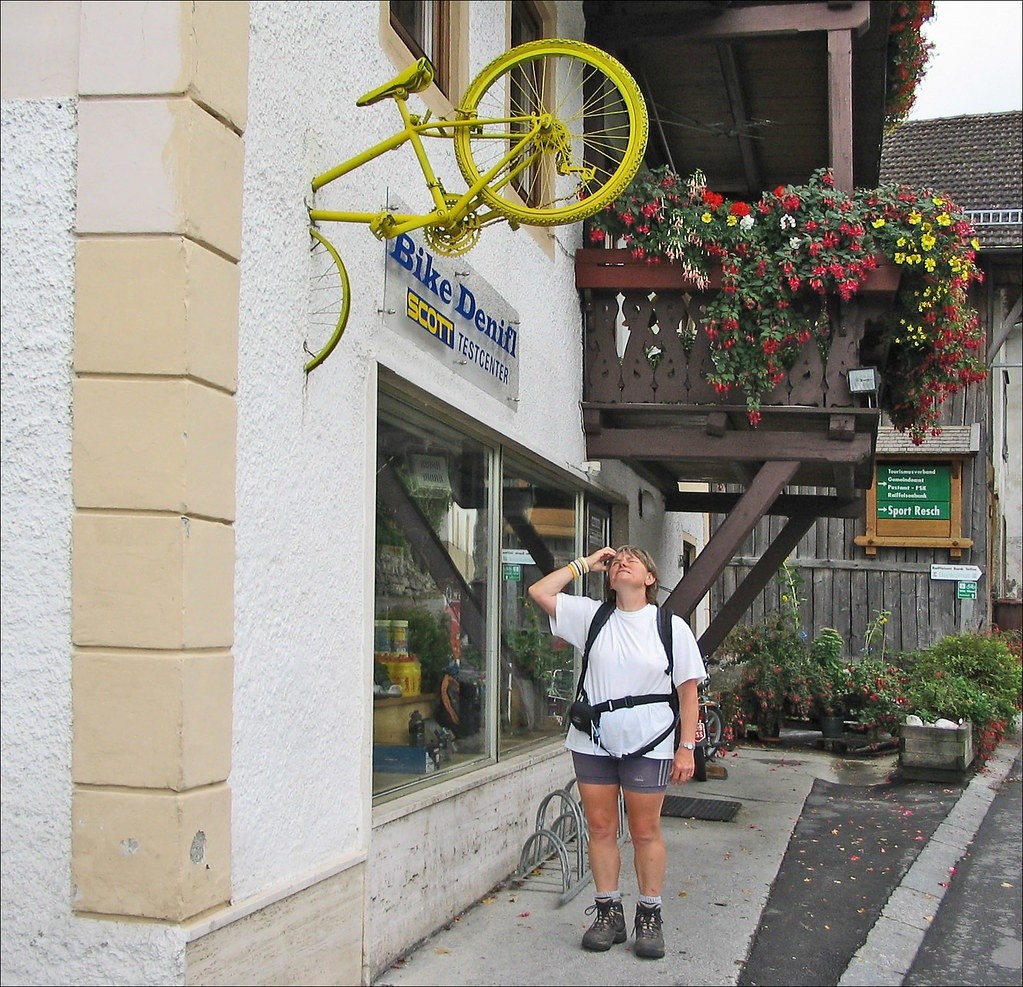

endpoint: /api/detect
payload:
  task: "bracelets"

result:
[568,557,589,580]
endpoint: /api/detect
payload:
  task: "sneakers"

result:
[582,896,627,951]
[631,902,665,958]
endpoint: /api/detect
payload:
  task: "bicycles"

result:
[307,37,650,371]
[696,655,724,763]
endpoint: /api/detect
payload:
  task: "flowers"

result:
[705,619,912,750]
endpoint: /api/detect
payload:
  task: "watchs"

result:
[679,743,695,749]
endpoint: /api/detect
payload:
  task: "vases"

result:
[817,716,846,739]
[755,711,781,740]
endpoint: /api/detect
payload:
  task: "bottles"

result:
[374,620,422,695]
[408,710,424,748]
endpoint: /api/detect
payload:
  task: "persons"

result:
[528,545,707,957]
